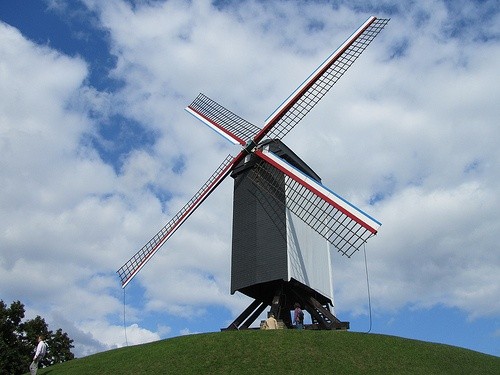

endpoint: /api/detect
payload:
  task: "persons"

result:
[31,335,47,365]
[292,302,305,329]
[265,311,278,329]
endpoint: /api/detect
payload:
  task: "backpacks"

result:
[299,312,304,321]
